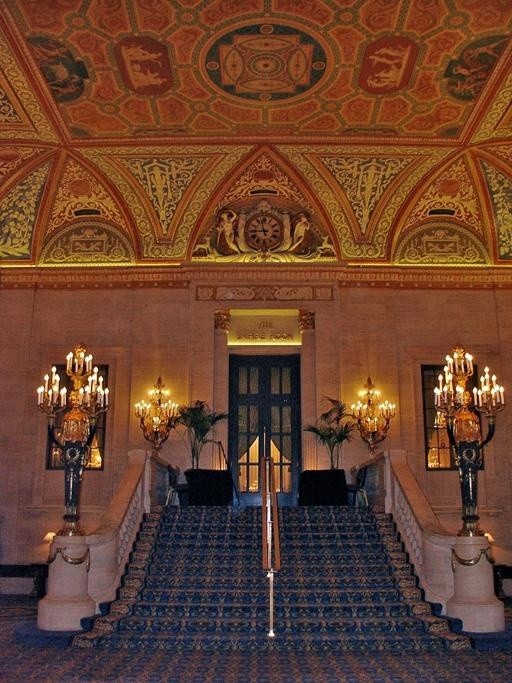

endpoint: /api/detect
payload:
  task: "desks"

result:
[299,470,348,505]
[184,468,234,506]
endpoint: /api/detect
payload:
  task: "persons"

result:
[288,211,310,250]
[217,209,240,252]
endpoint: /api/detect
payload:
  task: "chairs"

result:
[165,464,191,507]
[346,467,370,506]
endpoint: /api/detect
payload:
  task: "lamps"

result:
[430,343,507,537]
[36,342,110,536]
[134,377,179,451]
[350,376,397,456]
